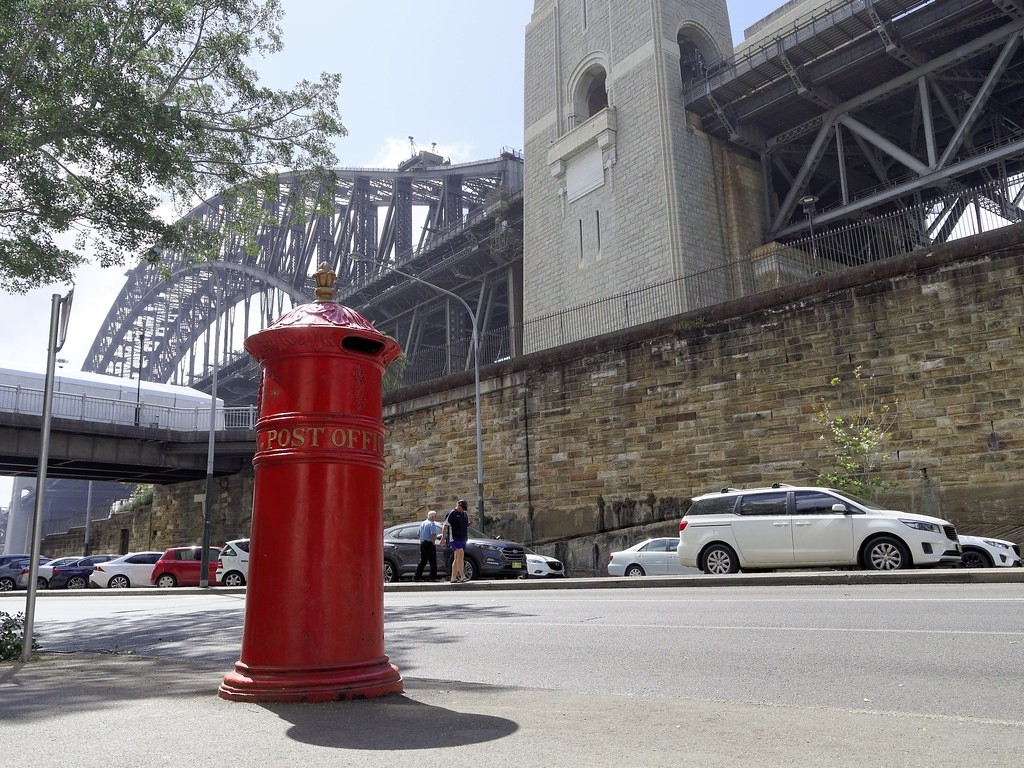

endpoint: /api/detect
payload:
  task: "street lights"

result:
[200,258,225,587]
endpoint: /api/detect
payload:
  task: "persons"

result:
[441,500,471,583]
[413,511,439,581]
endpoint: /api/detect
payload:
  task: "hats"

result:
[458,499,469,511]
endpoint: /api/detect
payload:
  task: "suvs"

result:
[0,553,45,566]
[381,521,529,584]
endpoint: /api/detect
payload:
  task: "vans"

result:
[677,481,963,572]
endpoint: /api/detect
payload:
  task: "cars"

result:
[53,555,123,590]
[518,545,565,580]
[90,552,169,591]
[0,557,54,592]
[151,546,230,589]
[215,538,250,588]
[17,556,89,589]
[931,531,1023,569]
[606,537,742,579]
